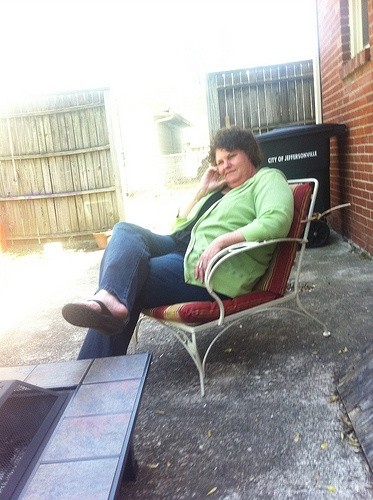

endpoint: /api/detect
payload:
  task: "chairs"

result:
[124,178,332,398]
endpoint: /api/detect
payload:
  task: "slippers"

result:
[62,299,125,334]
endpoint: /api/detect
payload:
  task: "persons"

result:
[62,123,294,360]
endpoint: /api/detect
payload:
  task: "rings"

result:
[198,262,203,270]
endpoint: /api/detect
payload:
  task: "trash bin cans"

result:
[253,123,345,247]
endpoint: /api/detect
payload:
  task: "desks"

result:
[0,353,155,500]
